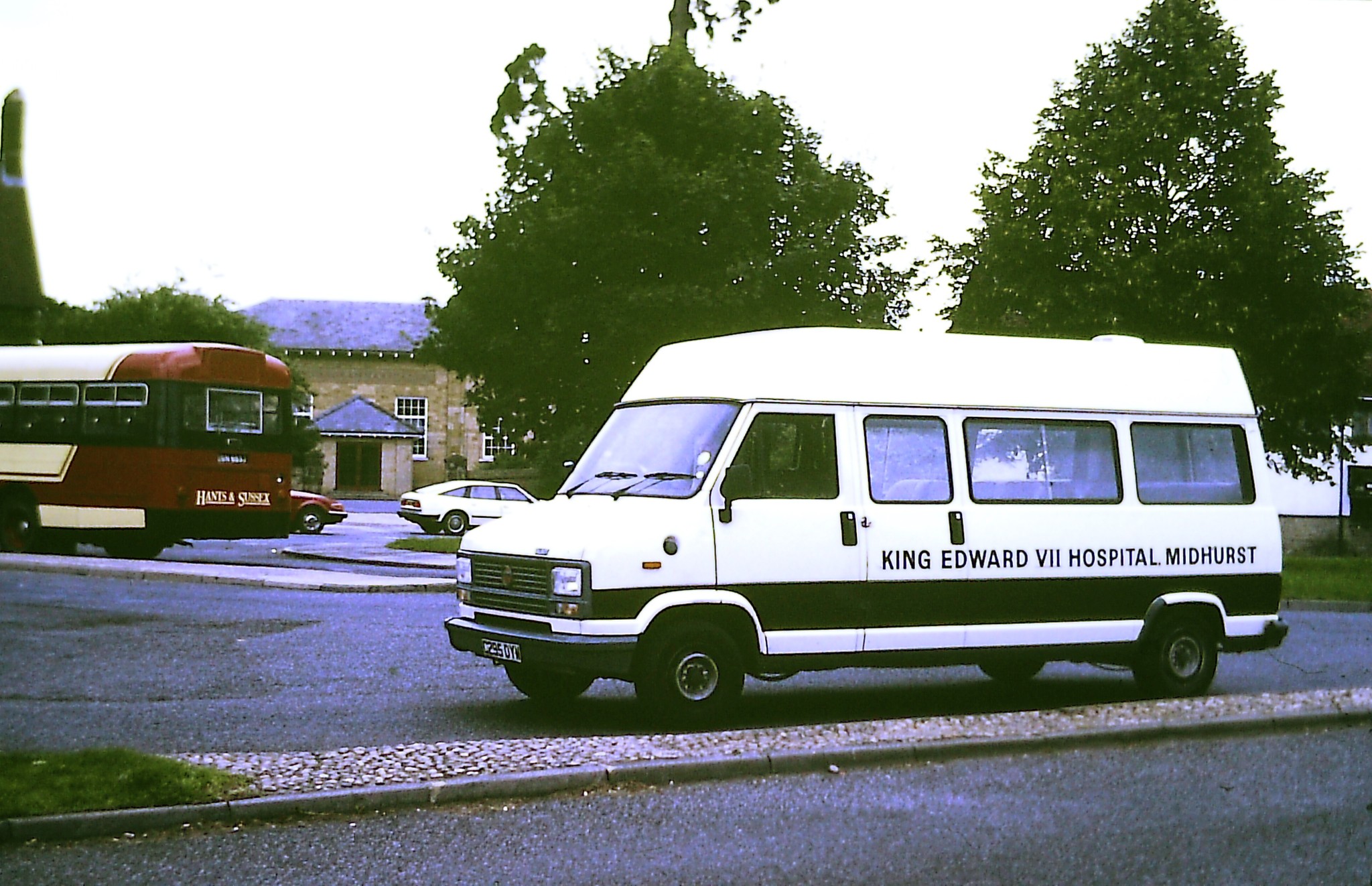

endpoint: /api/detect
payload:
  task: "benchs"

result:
[885,480,1242,503]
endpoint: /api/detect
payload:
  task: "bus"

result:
[0,340,295,561]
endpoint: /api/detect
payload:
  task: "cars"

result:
[290,490,347,535]
[399,480,540,537]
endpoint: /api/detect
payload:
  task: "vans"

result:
[442,332,1289,739]
